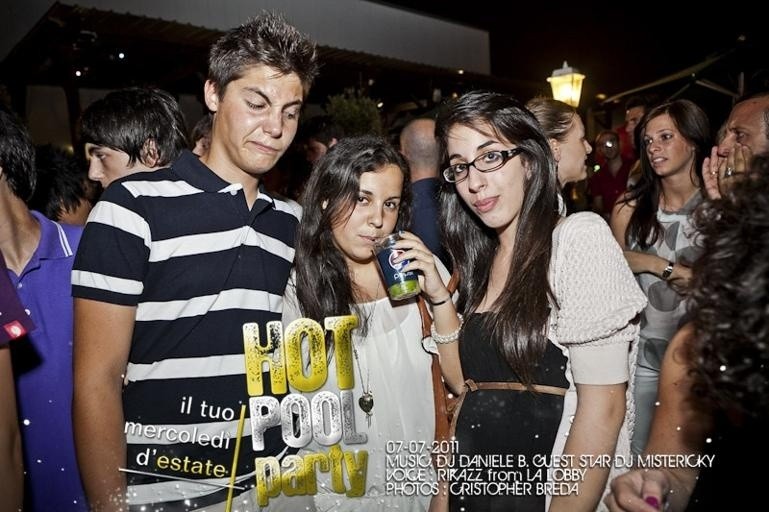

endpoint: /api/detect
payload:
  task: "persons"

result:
[1,8,769,511]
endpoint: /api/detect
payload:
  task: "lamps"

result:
[546,59,587,111]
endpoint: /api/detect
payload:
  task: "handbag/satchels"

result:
[415,290,450,476]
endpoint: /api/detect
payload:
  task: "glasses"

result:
[441,147,523,184]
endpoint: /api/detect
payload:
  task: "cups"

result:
[371,233,421,303]
